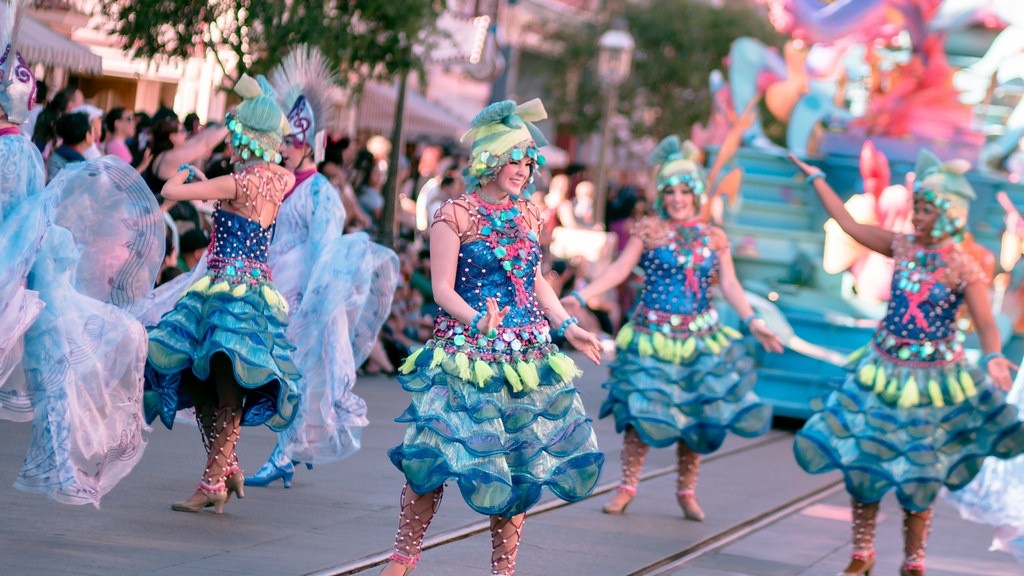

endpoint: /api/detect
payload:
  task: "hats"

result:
[71,105,103,120]
[225,73,283,164]
[649,135,706,220]
[181,228,210,253]
[912,149,976,237]
[460,98,549,199]
[266,42,345,162]
[0,0,38,124]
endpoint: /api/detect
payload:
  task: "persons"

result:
[23,82,650,378]
[375,95,605,576]
[144,134,399,489]
[790,154,1024,576]
[138,73,303,516]
[559,135,785,521]
[0,44,166,508]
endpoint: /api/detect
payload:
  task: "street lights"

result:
[596,27,634,228]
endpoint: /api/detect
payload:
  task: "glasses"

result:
[121,117,133,122]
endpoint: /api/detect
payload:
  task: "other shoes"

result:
[376,558,414,576]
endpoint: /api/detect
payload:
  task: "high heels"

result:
[291,446,314,470]
[837,555,877,576]
[900,564,926,576]
[677,496,704,521]
[603,492,634,513]
[224,469,245,499]
[172,489,227,514]
[244,460,296,489]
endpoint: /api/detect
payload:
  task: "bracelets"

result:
[572,291,585,307]
[806,172,827,184]
[557,316,579,337]
[743,313,760,328]
[983,353,1005,365]
[471,310,487,334]
[178,164,194,181]
